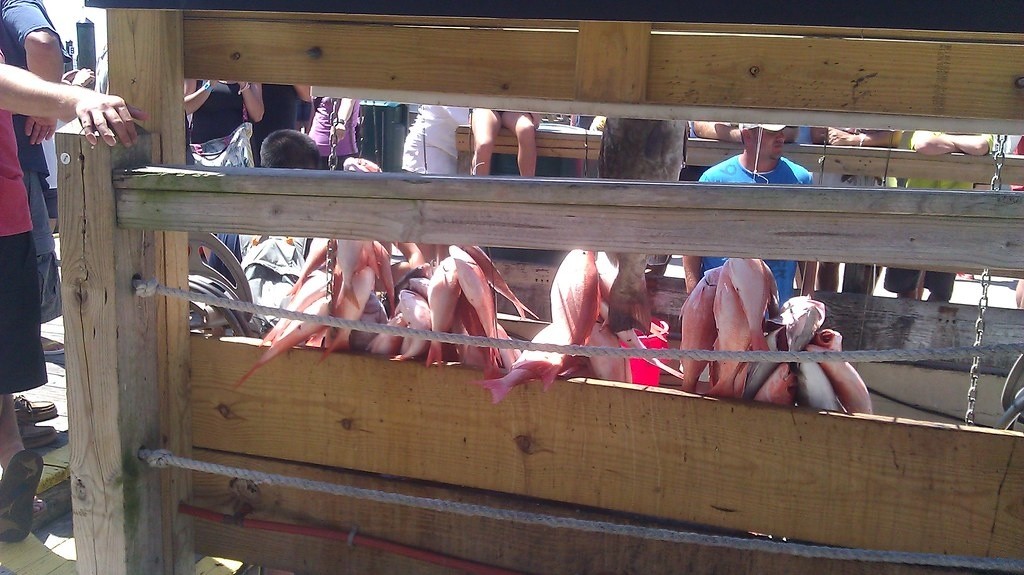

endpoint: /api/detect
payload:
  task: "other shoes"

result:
[0,449,44,542]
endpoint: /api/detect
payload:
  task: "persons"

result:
[0,0,66,443]
[467,108,547,187]
[61,68,94,88]
[682,120,817,330]
[1006,133,1023,309]
[688,121,801,186]
[402,102,472,179]
[298,90,363,176]
[236,130,426,343]
[884,126,997,301]
[806,123,906,294]
[180,79,264,174]
[0,47,151,523]
[247,81,312,167]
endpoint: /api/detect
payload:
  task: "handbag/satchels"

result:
[187,121,253,170]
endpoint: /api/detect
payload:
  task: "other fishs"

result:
[230,156,874,418]
[596,116,687,337]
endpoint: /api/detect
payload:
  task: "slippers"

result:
[40,337,65,355]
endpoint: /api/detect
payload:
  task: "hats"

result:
[739,122,786,132]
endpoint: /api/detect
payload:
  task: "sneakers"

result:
[11,394,58,421]
[15,419,57,449]
